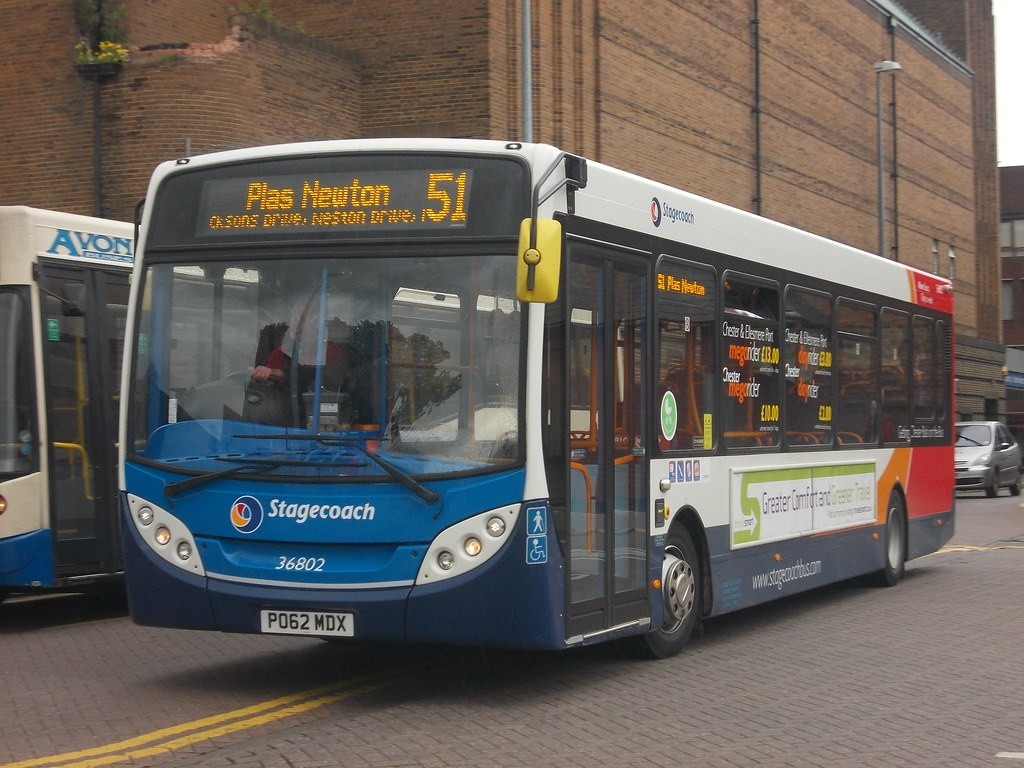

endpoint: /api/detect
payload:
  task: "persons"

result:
[246,291,349,393]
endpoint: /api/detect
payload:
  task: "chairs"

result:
[622,365,920,450]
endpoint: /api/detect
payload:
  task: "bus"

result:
[0,204,690,601]
[118,136,959,656]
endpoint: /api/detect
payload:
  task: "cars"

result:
[954,421,1022,497]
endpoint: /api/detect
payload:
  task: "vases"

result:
[75,63,121,81]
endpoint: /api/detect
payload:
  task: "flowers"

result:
[73,39,129,64]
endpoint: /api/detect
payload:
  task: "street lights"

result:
[874,59,907,260]
